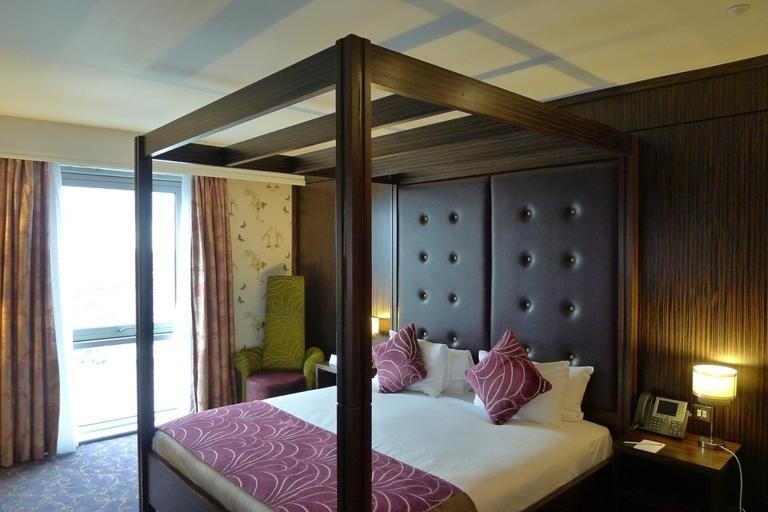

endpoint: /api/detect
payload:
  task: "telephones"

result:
[632,391,689,438]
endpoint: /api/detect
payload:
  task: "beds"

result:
[134,33,640,511]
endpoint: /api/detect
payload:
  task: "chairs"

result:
[231,276,326,402]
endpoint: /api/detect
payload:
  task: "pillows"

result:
[372,320,594,425]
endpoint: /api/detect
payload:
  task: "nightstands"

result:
[315,362,375,389]
[612,426,745,512]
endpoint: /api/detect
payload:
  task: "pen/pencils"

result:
[624,441,662,446]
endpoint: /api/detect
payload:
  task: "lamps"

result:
[692,365,738,451]
[370,317,379,335]
